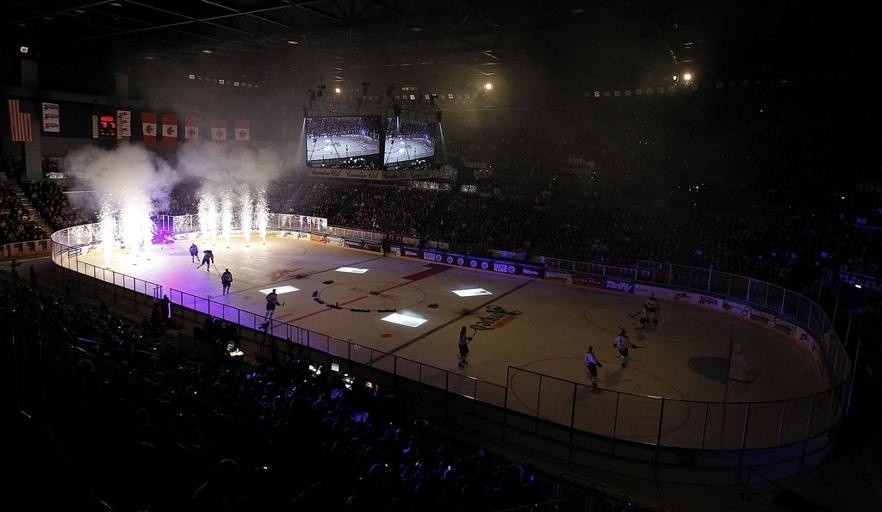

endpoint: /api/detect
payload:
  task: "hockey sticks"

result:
[627,307,644,318]
[465,327,476,345]
[211,263,220,276]
[273,301,285,306]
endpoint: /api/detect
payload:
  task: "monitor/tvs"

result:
[330,362,339,372]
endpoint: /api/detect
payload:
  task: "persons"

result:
[647,293,659,325]
[0,176,326,241]
[311,177,881,293]
[635,303,649,330]
[2,242,535,509]
[583,345,602,389]
[613,329,638,369]
